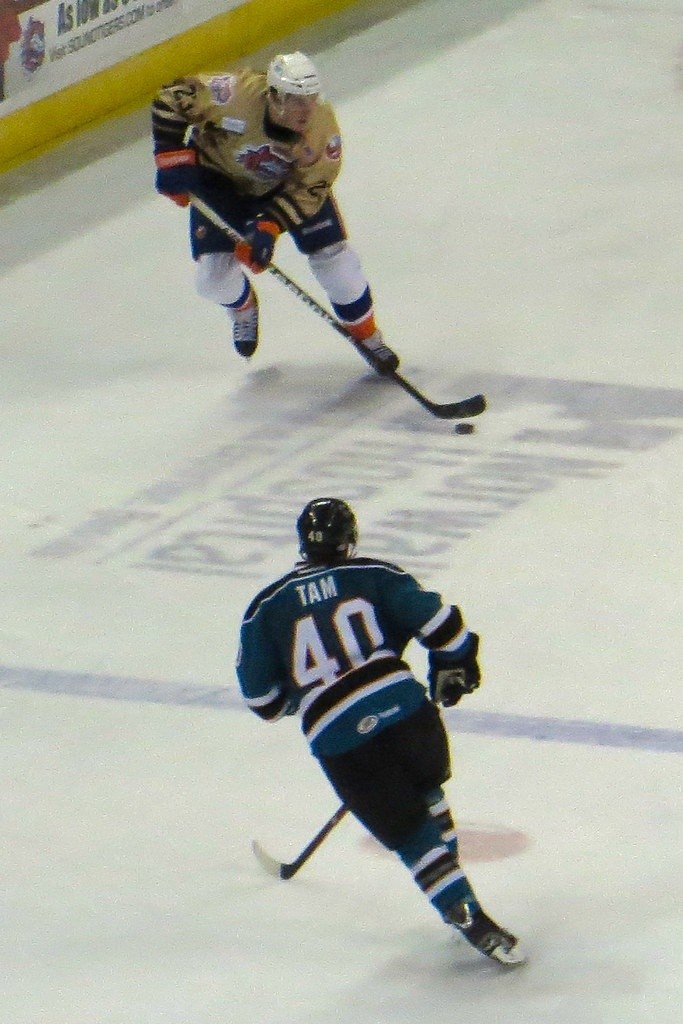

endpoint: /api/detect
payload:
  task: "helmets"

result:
[296,498,356,553]
[268,52,321,96]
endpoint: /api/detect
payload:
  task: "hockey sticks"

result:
[186,189,489,420]
[251,803,350,880]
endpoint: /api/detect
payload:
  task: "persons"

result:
[149,47,398,377]
[232,496,527,970]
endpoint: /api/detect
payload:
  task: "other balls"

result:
[455,422,475,435]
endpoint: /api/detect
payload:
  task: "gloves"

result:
[234,215,279,274]
[428,632,481,708]
[154,149,201,207]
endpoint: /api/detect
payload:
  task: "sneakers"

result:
[222,281,260,357]
[454,903,525,963]
[335,306,399,377]
